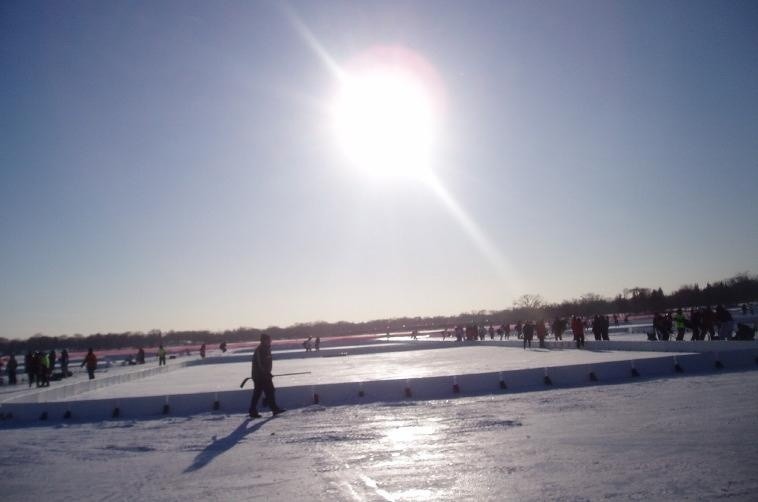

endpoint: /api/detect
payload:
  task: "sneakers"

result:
[273,407,287,416]
[250,412,262,418]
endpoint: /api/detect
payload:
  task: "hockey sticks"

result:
[240,372,311,388]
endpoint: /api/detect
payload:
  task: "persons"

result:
[125,346,145,365]
[410,329,417,340]
[220,342,227,353]
[304,336,320,352]
[200,344,206,360]
[442,312,629,351]
[0,346,68,388]
[248,333,288,418]
[80,348,97,380]
[156,345,166,366]
[653,302,758,341]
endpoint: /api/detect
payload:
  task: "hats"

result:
[260,334,271,343]
